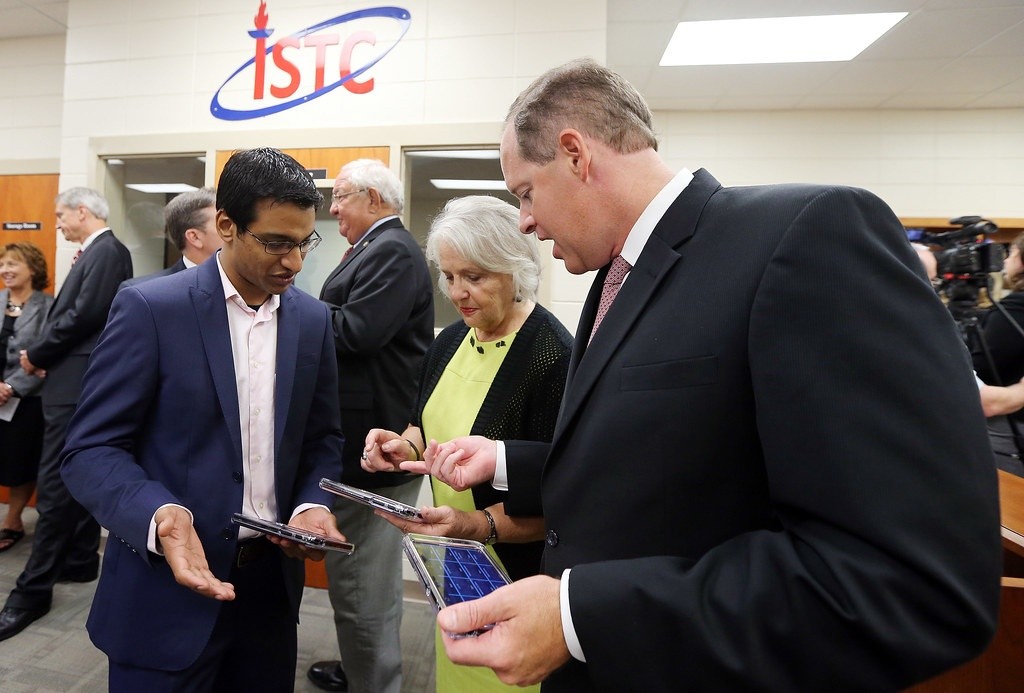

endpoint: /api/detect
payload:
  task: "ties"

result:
[340,247,354,264]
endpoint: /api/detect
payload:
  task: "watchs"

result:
[482,509,497,545]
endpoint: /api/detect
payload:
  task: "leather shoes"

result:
[0,590,51,641]
[307,660,350,693]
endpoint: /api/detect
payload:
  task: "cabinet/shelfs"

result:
[899,216,1024,308]
[905,467,1024,692]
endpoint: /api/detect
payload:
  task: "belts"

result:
[234,544,256,568]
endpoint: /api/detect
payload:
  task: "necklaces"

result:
[7,301,24,312]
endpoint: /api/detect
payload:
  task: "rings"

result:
[363,450,367,459]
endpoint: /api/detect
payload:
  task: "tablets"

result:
[230,513,355,556]
[319,478,425,524]
[401,533,514,639]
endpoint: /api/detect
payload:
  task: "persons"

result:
[362,196,574,693]
[118,187,223,294]
[399,61,1002,693]
[307,158,435,693]
[0,241,56,553]
[58,147,346,693]
[910,231,1024,478]
[0,187,134,643]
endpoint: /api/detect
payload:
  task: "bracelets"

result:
[406,439,420,461]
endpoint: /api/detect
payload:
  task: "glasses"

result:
[331,188,370,203]
[245,227,322,255]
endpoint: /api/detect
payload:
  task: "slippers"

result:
[0,528,25,553]
